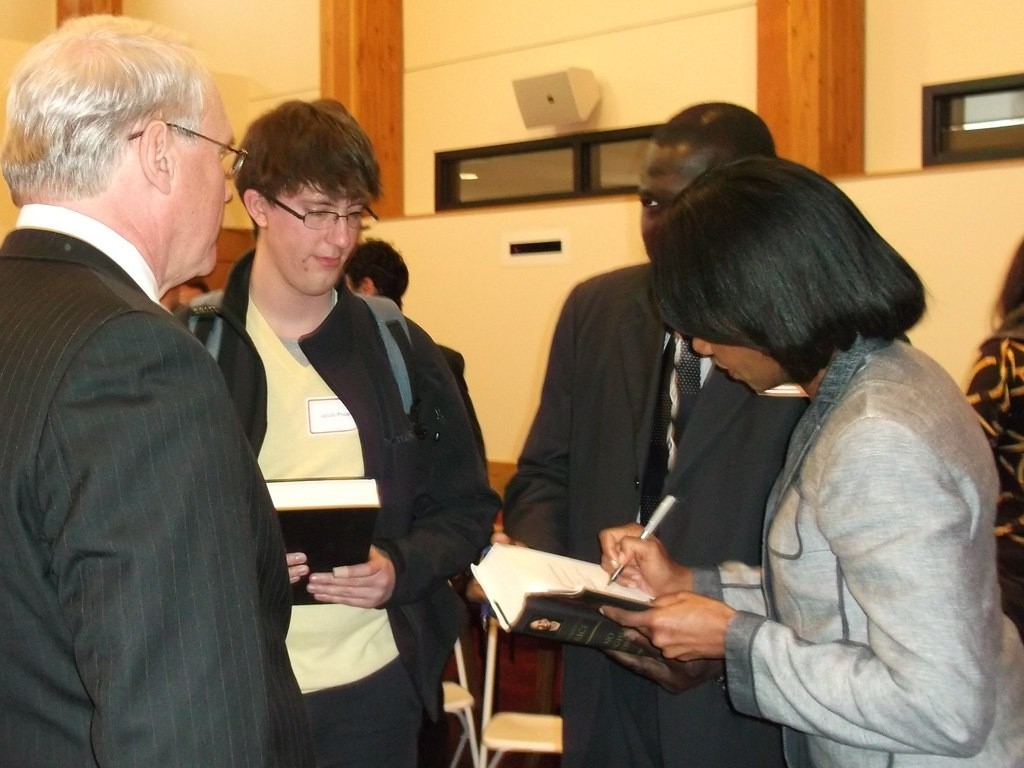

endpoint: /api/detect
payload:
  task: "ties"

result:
[641,328,701,543]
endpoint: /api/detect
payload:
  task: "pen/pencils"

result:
[607,493,679,589]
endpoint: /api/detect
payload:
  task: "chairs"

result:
[442,606,564,768]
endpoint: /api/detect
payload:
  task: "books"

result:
[265,477,381,605]
[471,543,664,658]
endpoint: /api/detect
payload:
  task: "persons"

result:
[493,101,812,768]
[965,236,1024,622]
[176,101,493,767]
[593,158,1024,768]
[162,278,211,306]
[0,13,312,768]
[347,242,484,460]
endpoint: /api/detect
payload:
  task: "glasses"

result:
[262,189,378,231]
[130,123,248,180]
